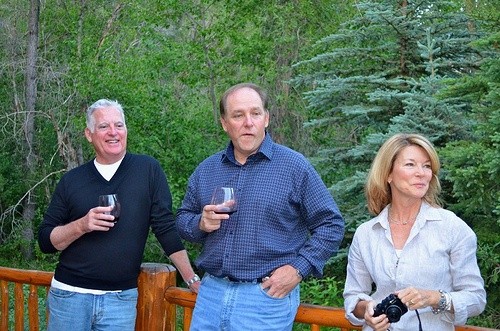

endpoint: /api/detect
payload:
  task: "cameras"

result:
[373,294,408,324]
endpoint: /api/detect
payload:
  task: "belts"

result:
[229,278,262,283]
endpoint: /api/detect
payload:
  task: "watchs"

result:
[186,274,200,286]
[296,269,303,278]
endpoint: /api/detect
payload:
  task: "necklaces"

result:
[389,216,416,225]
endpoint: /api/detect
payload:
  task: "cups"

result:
[99,194,120,225]
[210,187,238,215]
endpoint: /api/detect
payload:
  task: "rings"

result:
[409,300,414,305]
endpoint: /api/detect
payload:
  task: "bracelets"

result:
[432,290,446,315]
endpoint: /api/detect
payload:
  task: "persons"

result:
[175,83,345,331]
[342,133,487,331]
[37,99,202,331]
